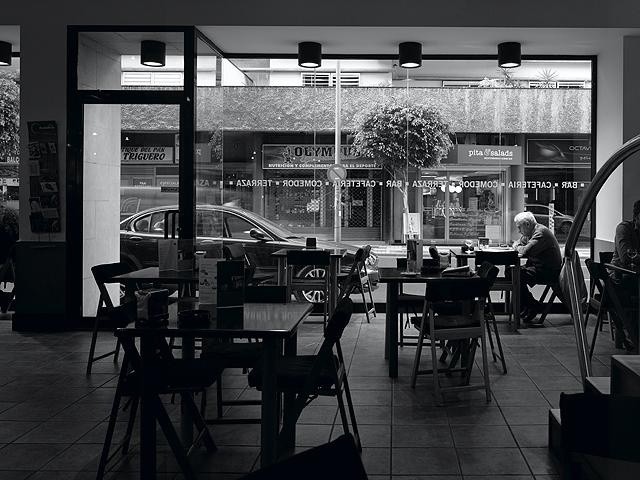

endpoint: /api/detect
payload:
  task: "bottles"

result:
[334,245,340,254]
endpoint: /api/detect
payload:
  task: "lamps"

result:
[399,42,424,68]
[497,42,523,68]
[142,39,168,66]
[0,40,17,67]
[296,43,323,68]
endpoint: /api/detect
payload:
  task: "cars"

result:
[524,204,575,235]
[121,206,382,310]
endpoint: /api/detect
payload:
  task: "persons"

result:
[514,211,562,321]
[606,199,640,349]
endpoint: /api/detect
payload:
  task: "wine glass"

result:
[465,239,473,255]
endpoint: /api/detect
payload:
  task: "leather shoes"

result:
[520,305,531,318]
[522,303,542,323]
